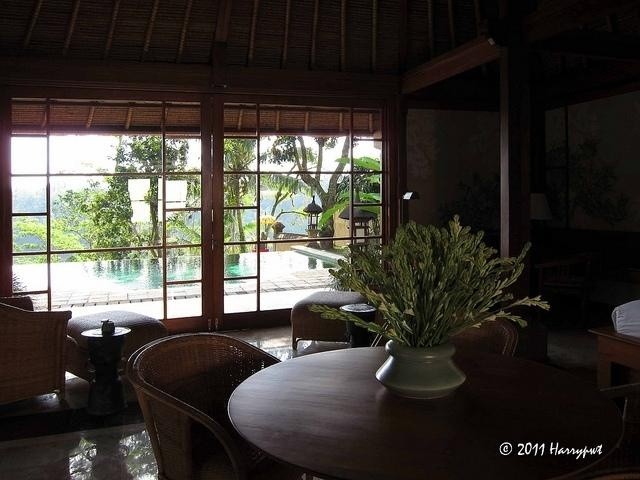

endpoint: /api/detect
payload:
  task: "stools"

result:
[67,310,167,385]
[291,290,368,351]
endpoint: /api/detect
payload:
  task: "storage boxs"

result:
[588,326,639,400]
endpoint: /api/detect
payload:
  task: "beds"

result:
[227,346,625,478]
[340,303,377,348]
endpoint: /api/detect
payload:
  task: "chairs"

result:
[0,296,72,407]
[125,332,314,480]
[80,327,133,416]
[454,311,519,358]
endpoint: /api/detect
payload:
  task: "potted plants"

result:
[308,213,552,400]
